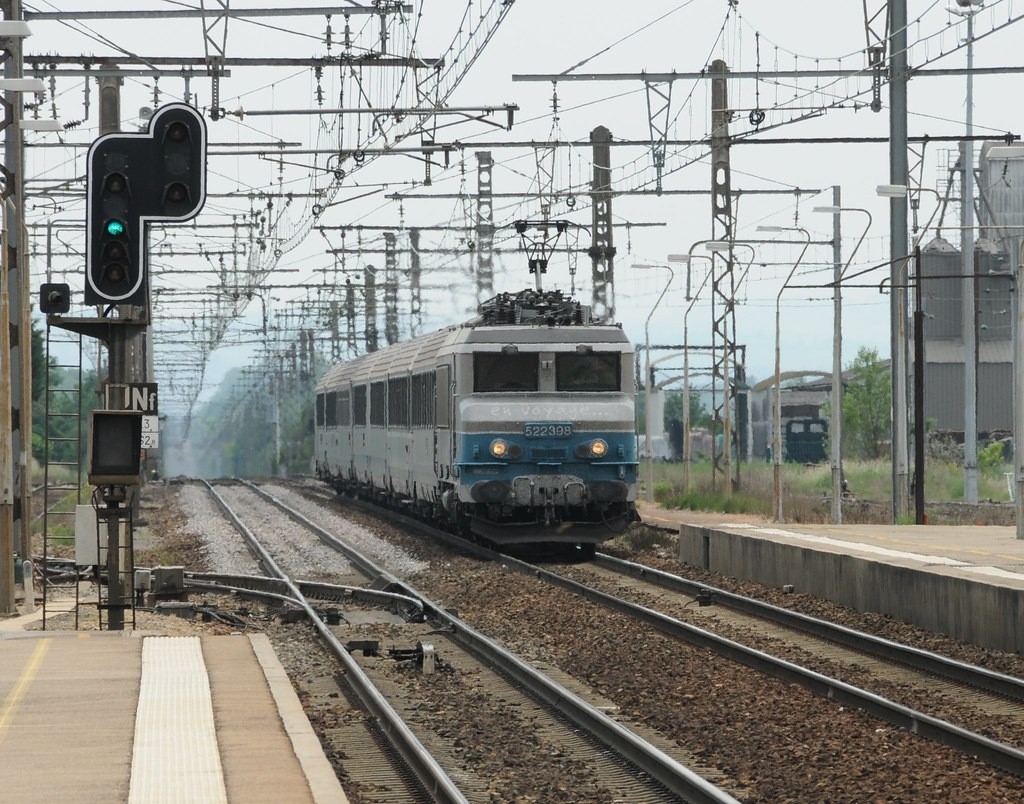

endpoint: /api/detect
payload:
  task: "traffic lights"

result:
[39,283,70,314]
[85,101,208,307]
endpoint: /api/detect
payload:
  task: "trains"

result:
[310,284,643,563]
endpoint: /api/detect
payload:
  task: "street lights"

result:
[876,184,940,525]
[754,224,810,528]
[810,203,873,526]
[667,254,715,500]
[707,240,755,506]
[630,262,675,505]
